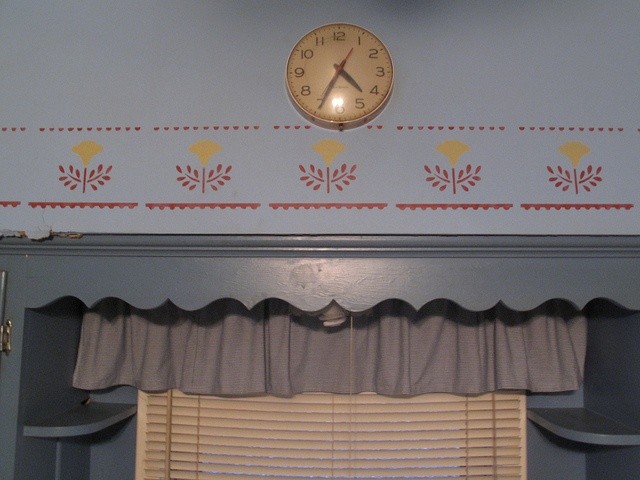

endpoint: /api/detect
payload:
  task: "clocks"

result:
[285,22,395,131]
